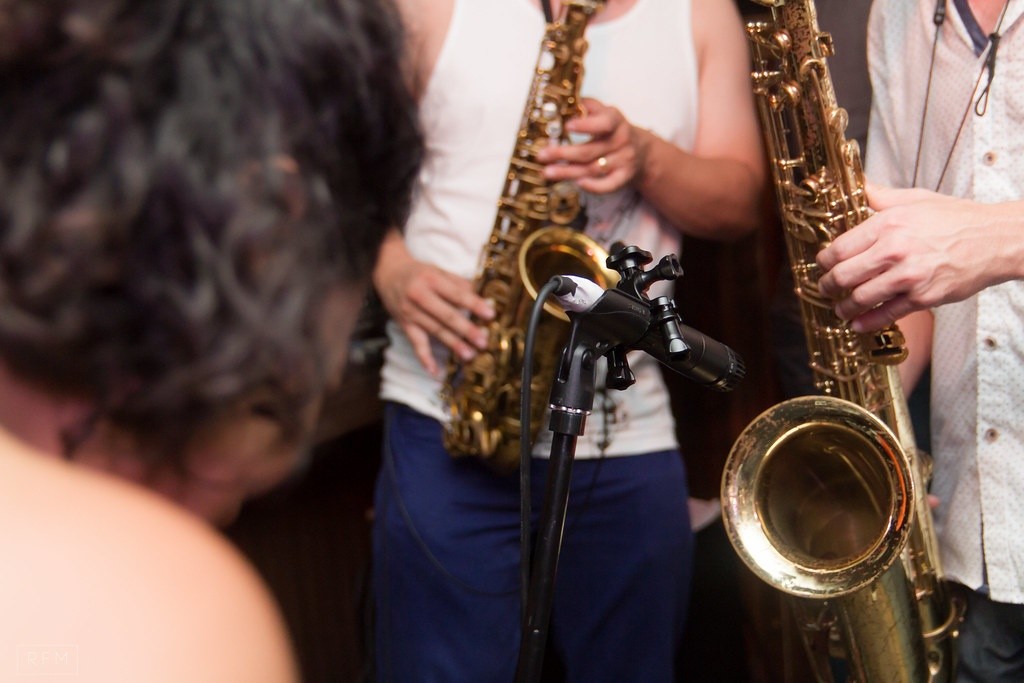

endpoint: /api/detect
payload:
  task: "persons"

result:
[816,0,1024,683]
[0,0,432,683]
[370,1,769,683]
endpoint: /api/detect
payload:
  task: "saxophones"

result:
[444,0,621,469]
[719,0,964,683]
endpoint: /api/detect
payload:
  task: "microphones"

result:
[550,274,746,395]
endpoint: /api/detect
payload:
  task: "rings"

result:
[595,157,607,174]
